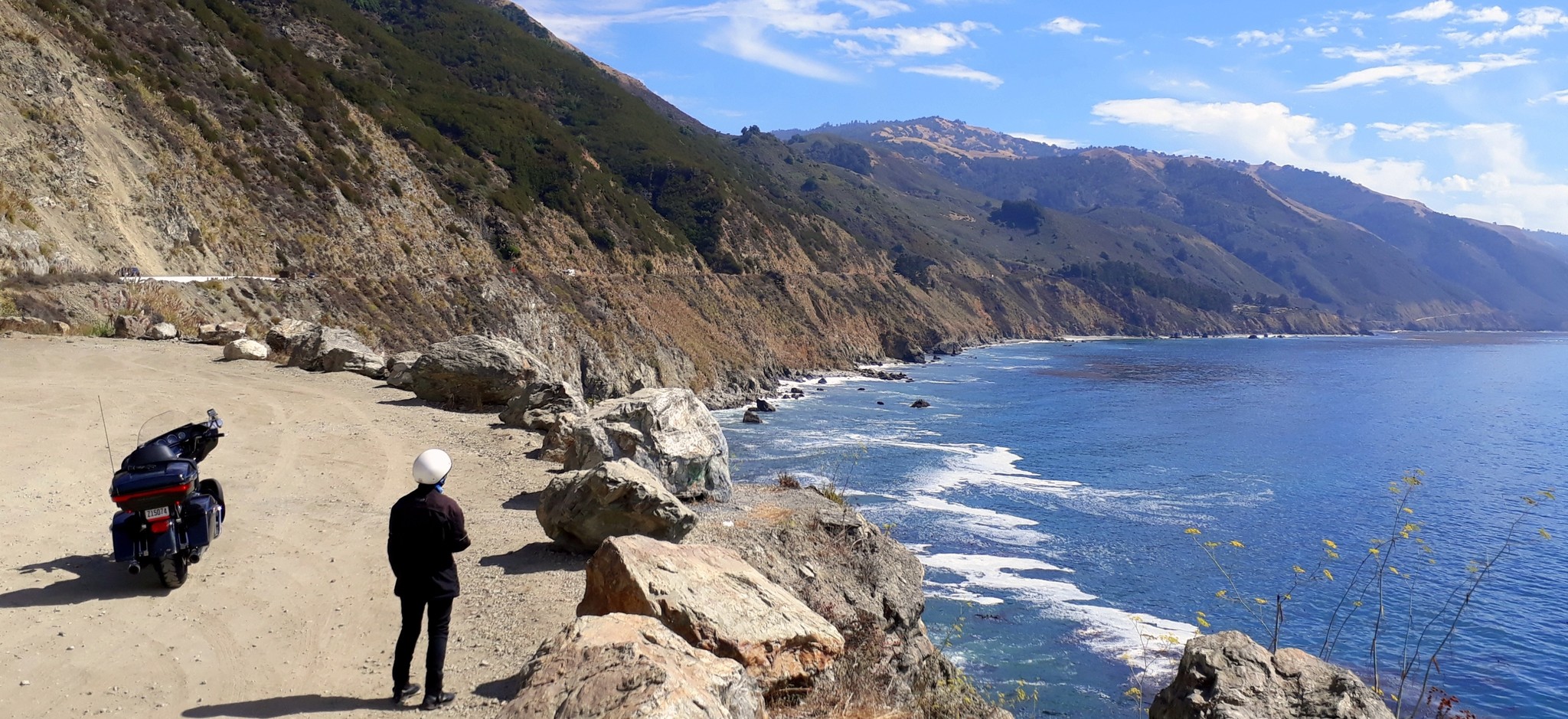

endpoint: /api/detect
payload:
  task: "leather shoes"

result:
[393,683,421,704]
[423,690,455,709]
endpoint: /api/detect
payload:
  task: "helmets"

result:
[412,448,452,493]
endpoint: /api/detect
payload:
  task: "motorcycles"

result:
[108,407,226,590]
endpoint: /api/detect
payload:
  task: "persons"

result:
[387,449,471,710]
